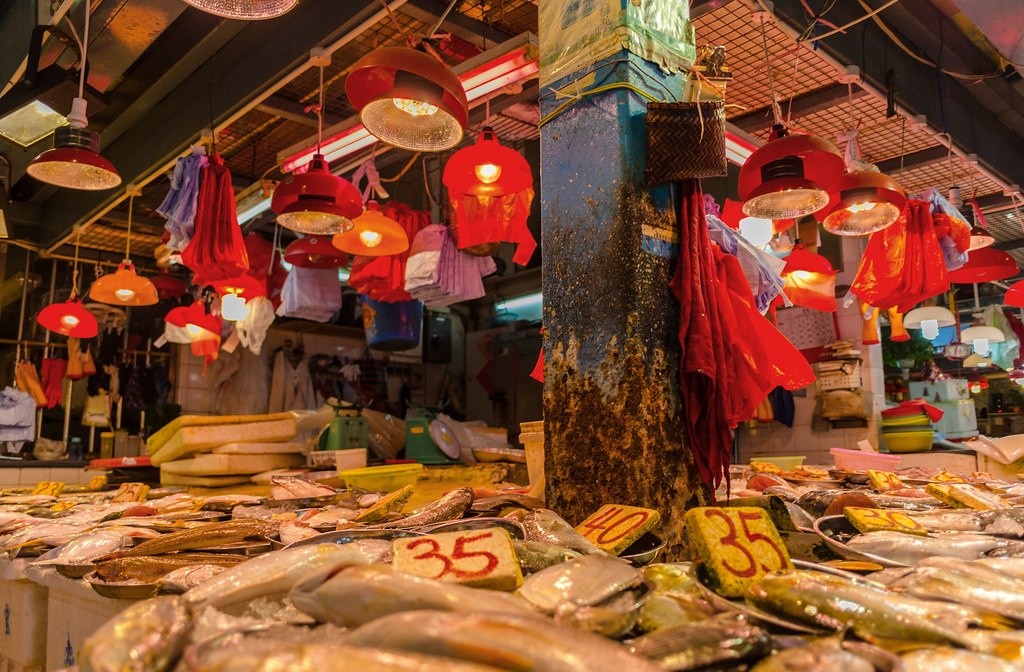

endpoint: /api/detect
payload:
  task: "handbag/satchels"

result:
[81,388,112,427]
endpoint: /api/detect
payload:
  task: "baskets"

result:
[819,360,861,390]
[646,100,727,186]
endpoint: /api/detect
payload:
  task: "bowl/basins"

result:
[830,447,902,472]
[881,400,934,452]
[750,456,806,472]
[339,463,422,496]
[311,449,335,468]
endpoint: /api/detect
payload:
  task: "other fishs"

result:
[0,472,1024,672]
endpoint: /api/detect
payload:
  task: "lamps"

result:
[1004,280,1024,308]
[236,180,281,226]
[963,352,993,368]
[967,370,989,393]
[24,0,121,191]
[443,1,533,193]
[0,64,111,148]
[944,248,1020,283]
[280,233,349,270]
[960,309,1005,354]
[813,67,904,236]
[903,297,955,340]
[331,148,409,257]
[277,31,538,176]
[738,1,845,220]
[89,193,158,307]
[932,132,996,250]
[35,231,97,339]
[149,267,186,299]
[270,66,364,233]
[346,37,469,151]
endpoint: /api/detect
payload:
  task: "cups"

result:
[336,448,367,474]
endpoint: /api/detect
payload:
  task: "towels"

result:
[665,186,740,509]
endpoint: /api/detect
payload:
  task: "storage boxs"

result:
[519,421,545,497]
[0,557,135,672]
[909,378,979,444]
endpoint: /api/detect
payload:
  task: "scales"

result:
[315,397,375,461]
[404,410,467,466]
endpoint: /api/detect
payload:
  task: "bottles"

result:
[100,428,139,458]
[69,437,83,462]
[991,389,1024,413]
